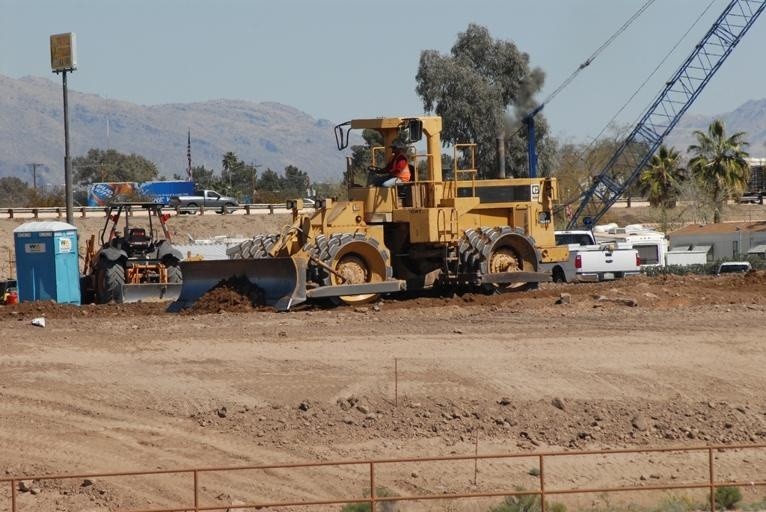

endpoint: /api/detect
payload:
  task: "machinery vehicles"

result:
[167,116,571,311]
[86,201,184,303]
[524,0,766,269]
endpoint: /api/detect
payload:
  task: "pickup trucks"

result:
[169,189,239,214]
[540,229,641,284]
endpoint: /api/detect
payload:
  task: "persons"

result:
[375,138,410,199]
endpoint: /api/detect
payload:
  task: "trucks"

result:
[85,180,203,211]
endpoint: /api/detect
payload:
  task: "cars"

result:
[302,197,316,208]
[717,261,752,275]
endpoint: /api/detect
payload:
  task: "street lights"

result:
[736,226,742,260]
